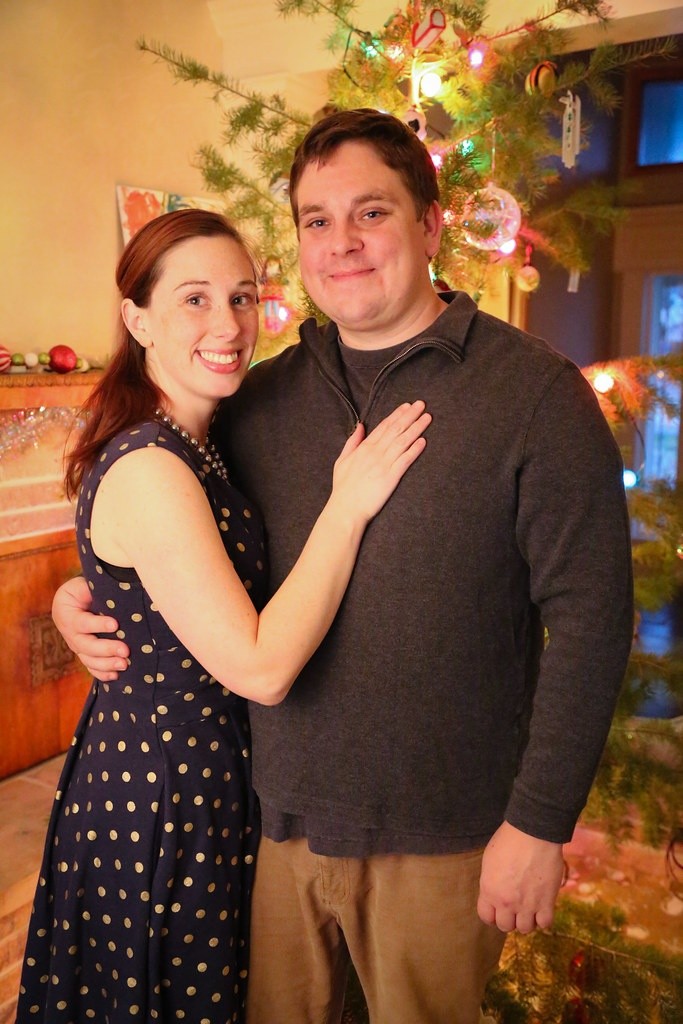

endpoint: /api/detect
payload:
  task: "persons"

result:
[15,208,432,1024]
[49,110,634,1024]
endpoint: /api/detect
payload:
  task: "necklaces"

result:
[152,405,229,483]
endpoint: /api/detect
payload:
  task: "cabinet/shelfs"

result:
[2,367,134,775]
